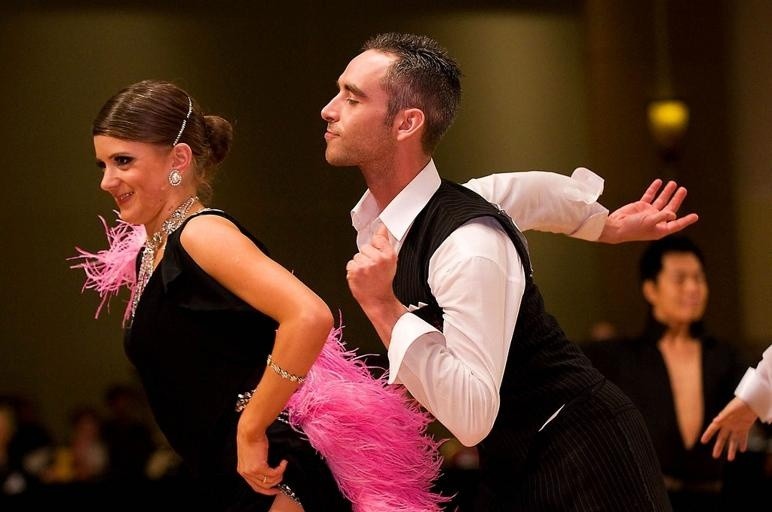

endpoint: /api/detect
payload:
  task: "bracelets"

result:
[267,355,308,386]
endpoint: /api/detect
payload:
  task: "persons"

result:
[699,347,772,463]
[92,74,351,512]
[0,385,162,511]
[576,232,747,510]
[319,30,702,510]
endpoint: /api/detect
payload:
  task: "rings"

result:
[262,476,266,485]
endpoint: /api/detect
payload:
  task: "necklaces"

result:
[121,195,204,328]
